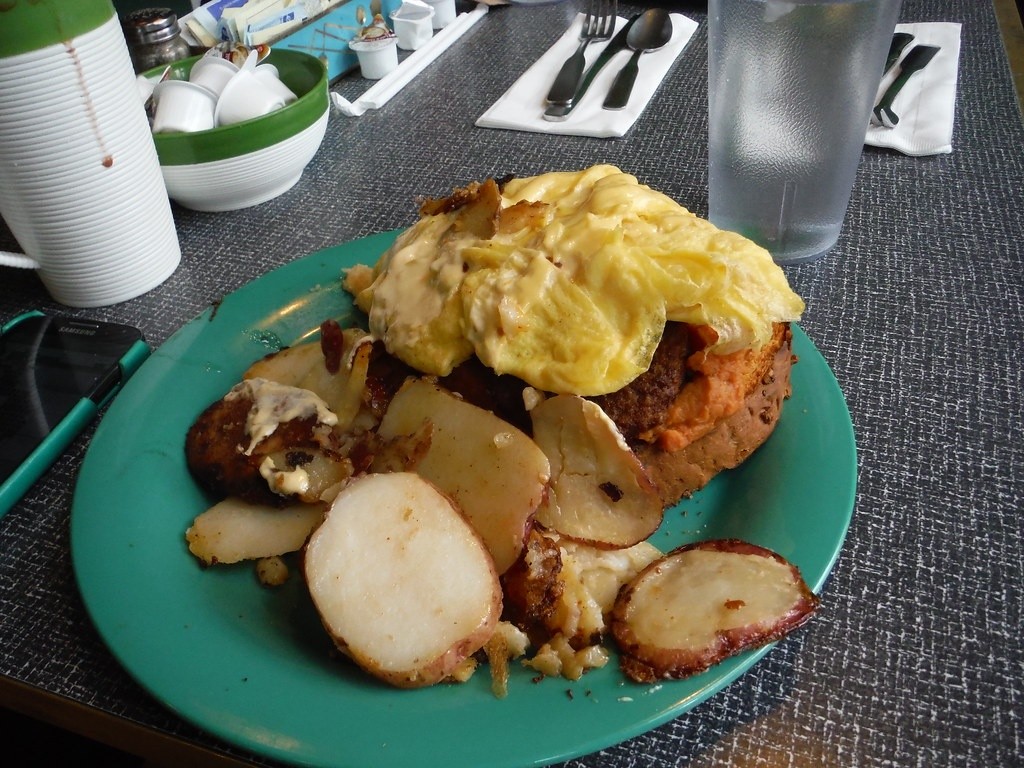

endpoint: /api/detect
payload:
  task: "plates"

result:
[68,227,858,768]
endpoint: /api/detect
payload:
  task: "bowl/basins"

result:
[136,48,331,212]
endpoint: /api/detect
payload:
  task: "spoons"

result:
[601,8,674,111]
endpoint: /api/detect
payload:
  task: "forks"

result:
[868,44,942,128]
[545,0,618,105]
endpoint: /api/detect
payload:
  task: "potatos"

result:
[185,322,818,687]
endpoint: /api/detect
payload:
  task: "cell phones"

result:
[0,307,153,519]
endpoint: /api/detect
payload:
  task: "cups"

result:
[706,0,901,267]
[0,0,182,309]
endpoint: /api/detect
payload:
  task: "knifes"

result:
[542,13,644,122]
